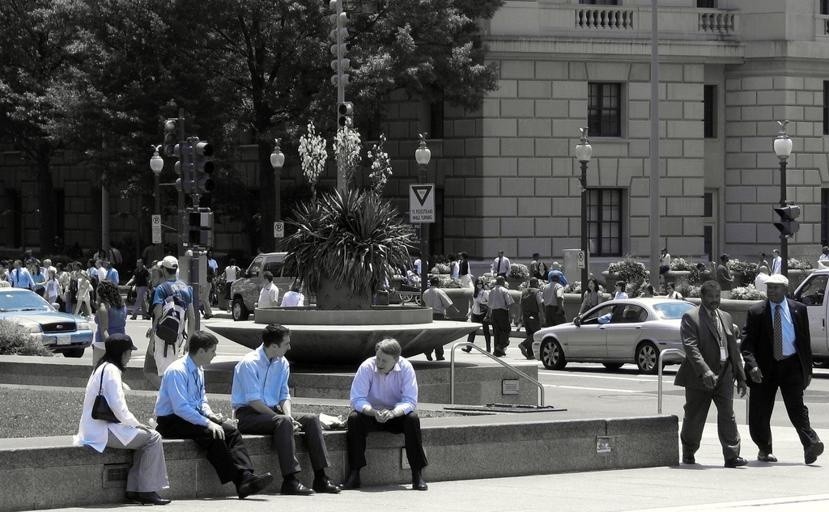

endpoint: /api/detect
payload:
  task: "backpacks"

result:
[156,283,188,357]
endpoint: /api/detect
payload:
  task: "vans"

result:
[228,252,317,321]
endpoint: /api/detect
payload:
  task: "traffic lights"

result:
[773,205,800,235]
[339,102,354,131]
[173,138,217,194]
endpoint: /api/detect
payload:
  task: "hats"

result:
[157,255,179,270]
[105,333,138,350]
[551,261,561,269]
[763,273,789,286]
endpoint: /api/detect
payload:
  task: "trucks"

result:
[791,268,829,369]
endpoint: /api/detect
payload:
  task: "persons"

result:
[222,258,241,299]
[819,246,829,261]
[717,254,734,290]
[756,252,771,275]
[95,259,107,282]
[459,252,468,275]
[660,248,671,273]
[280,279,304,307]
[93,249,109,261]
[258,271,279,308]
[665,282,683,300]
[103,259,119,286]
[231,324,340,495]
[30,264,46,296]
[423,277,460,361]
[203,260,213,319]
[65,264,72,314]
[56,263,68,312]
[754,266,770,293]
[518,277,545,359]
[71,261,82,313]
[207,249,218,277]
[697,263,706,284]
[577,278,600,316]
[640,285,655,297]
[771,249,782,273]
[414,252,449,275]
[0,262,12,287]
[611,281,630,311]
[488,276,515,357]
[494,251,510,281]
[143,256,195,390]
[86,259,98,313]
[448,254,459,279]
[126,258,151,320]
[43,266,60,311]
[548,262,567,287]
[74,270,94,320]
[76,333,171,506]
[541,273,567,326]
[740,274,824,464]
[11,260,35,290]
[674,280,748,468]
[94,280,127,341]
[461,278,490,353]
[460,270,474,312]
[156,330,274,500]
[530,253,539,277]
[338,338,428,491]
[43,259,51,281]
[26,259,34,272]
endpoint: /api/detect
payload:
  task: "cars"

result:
[0,281,94,358]
[532,298,742,374]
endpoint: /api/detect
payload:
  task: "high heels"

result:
[137,492,171,505]
[126,491,140,501]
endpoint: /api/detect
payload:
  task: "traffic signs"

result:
[410,183,435,224]
[152,215,161,244]
[273,222,284,238]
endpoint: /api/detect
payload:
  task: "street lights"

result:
[576,127,591,306]
[269,138,285,251]
[773,120,793,278]
[415,132,431,307]
[150,143,164,261]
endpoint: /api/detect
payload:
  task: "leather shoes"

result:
[805,440,824,464]
[313,479,341,493]
[725,455,748,467]
[682,448,695,464]
[280,479,314,495]
[238,471,273,499]
[339,477,361,490]
[527,356,536,359]
[412,478,428,490]
[518,344,528,357]
[757,453,777,462]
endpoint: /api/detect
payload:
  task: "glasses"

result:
[477,282,483,285]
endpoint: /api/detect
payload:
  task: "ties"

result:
[772,260,777,272]
[772,305,784,361]
[497,260,500,272]
[710,311,718,332]
[16,269,19,284]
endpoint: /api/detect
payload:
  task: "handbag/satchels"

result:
[91,364,122,423]
[479,293,488,312]
